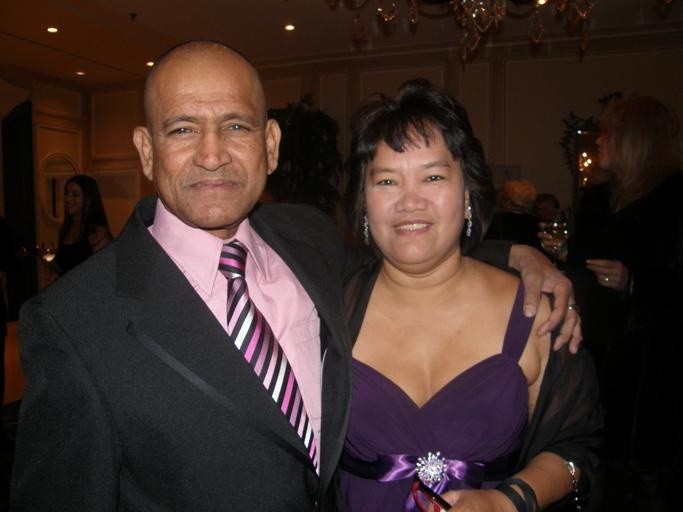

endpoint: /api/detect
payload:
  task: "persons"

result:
[537,94,683,512]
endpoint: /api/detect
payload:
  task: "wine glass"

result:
[41,241,56,280]
[540,216,569,274]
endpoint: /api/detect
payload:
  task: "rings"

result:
[605,275,608,282]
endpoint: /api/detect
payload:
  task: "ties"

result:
[218,240,318,476]
